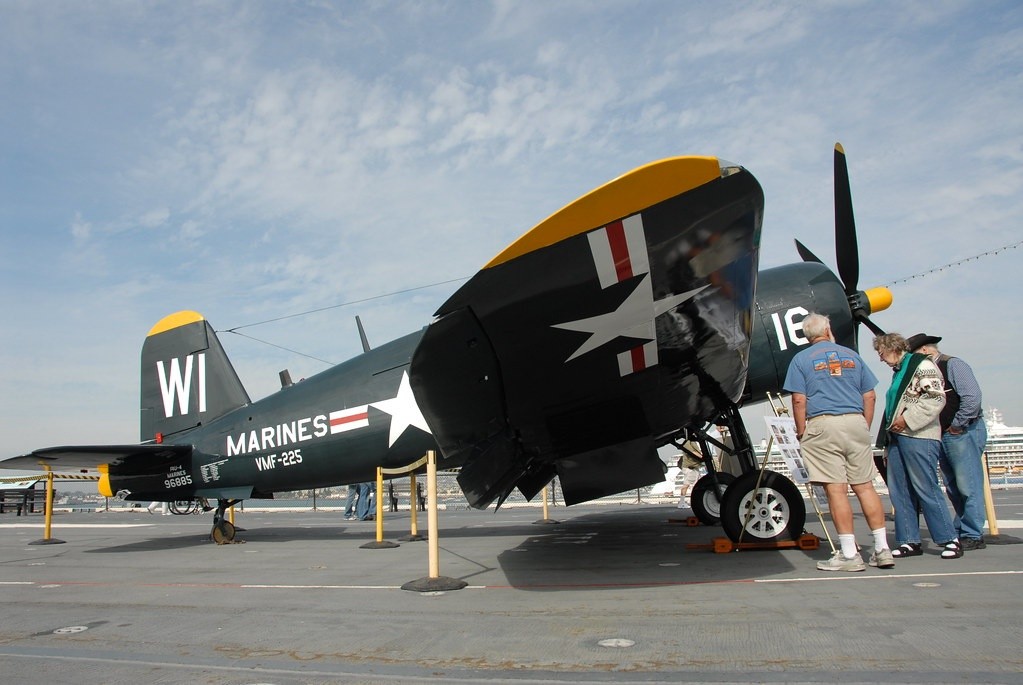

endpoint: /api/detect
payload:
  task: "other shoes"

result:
[147,508,153,515]
[344,515,357,521]
[163,512,170,515]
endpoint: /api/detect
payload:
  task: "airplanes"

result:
[0,142,892,546]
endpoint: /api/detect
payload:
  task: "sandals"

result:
[891,542,924,557]
[941,541,963,559]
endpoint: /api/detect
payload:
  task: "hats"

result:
[904,333,942,354]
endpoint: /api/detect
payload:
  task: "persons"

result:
[873,333,965,559]
[783,314,895,571]
[678,434,703,508]
[147,502,171,515]
[705,424,727,472]
[905,334,987,550]
[343,481,377,521]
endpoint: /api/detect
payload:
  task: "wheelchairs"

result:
[167,496,206,516]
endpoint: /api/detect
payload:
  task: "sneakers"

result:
[816,548,866,571]
[868,548,895,567]
[961,536,986,550]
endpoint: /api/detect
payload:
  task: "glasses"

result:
[877,350,884,358]
